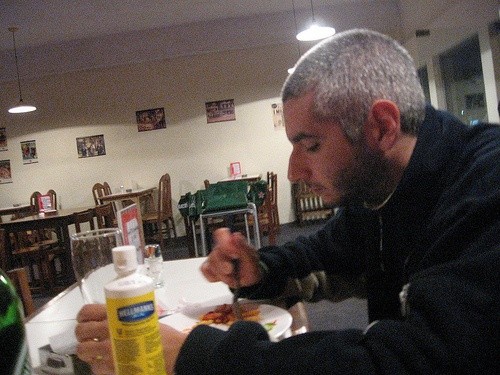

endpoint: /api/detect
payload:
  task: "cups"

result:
[70,227,124,360]
[144,244,166,288]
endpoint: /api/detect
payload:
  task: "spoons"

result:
[231,258,243,322]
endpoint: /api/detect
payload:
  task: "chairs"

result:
[92,173,177,252]
[179,172,280,259]
[4,268,35,318]
[29,188,57,216]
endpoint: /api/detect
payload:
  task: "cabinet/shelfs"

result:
[291,184,335,226]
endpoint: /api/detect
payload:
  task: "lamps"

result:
[296,0,336,42]
[8,27,37,115]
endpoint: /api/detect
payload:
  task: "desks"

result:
[97,186,157,215]
[21,257,235,375]
[0,208,96,285]
[0,204,33,220]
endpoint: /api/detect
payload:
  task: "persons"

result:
[138,111,165,130]
[75,29,500,375]
[82,143,104,156]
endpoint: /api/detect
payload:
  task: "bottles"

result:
[1,267,31,375]
[120,186,125,193]
[104,246,166,375]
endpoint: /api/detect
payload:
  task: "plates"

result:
[160,303,293,341]
